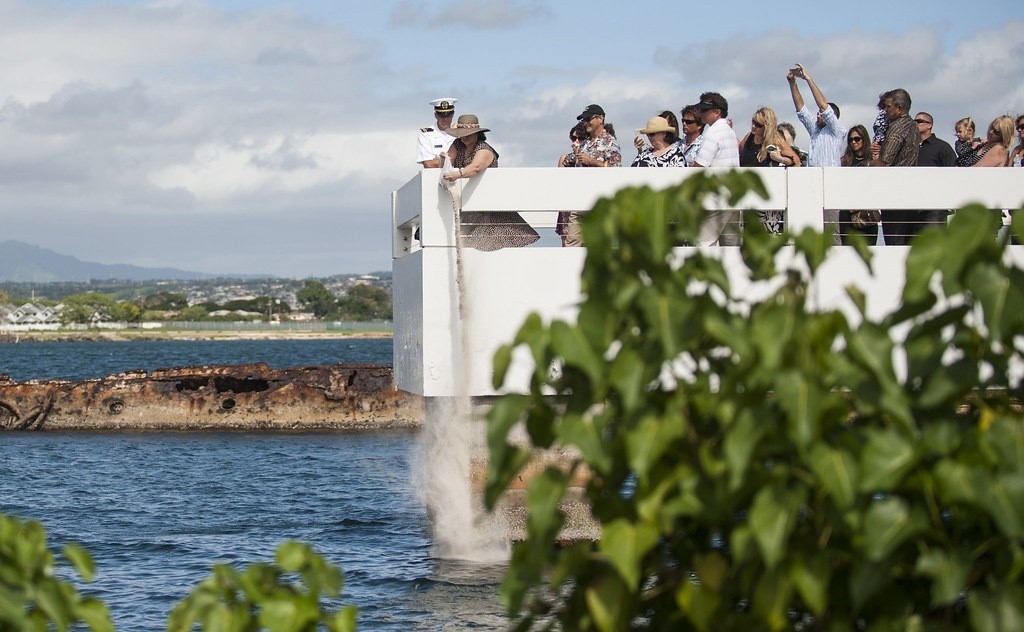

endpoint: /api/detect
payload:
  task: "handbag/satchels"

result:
[851,209,881,229]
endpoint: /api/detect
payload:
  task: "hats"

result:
[577,105,605,120]
[694,99,719,110]
[445,115,490,138]
[429,97,459,117]
[639,117,676,134]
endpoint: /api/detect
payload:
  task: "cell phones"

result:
[634,129,645,145]
[766,145,777,151]
[790,69,801,77]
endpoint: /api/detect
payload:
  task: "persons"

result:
[555,105,621,247]
[631,94,809,247]
[840,90,1024,246]
[416,98,539,252]
[786,63,846,246]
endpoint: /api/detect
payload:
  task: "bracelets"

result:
[459,170,463,177]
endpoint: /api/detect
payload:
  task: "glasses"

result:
[990,125,1002,137]
[681,118,699,125]
[1016,123,1024,130]
[913,118,930,123]
[581,115,600,123]
[646,132,664,137]
[751,119,763,129]
[571,135,587,142]
[699,108,715,114]
[849,136,863,143]
